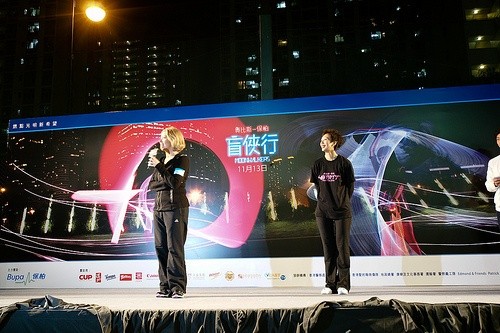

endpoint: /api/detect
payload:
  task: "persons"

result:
[147,127,190,298]
[370,130,421,255]
[308,129,355,295]
[484,132,500,228]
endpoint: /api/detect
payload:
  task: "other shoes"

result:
[156,289,172,297]
[337,287,348,295]
[173,290,184,298]
[321,287,332,294]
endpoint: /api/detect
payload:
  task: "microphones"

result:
[147,148,161,169]
